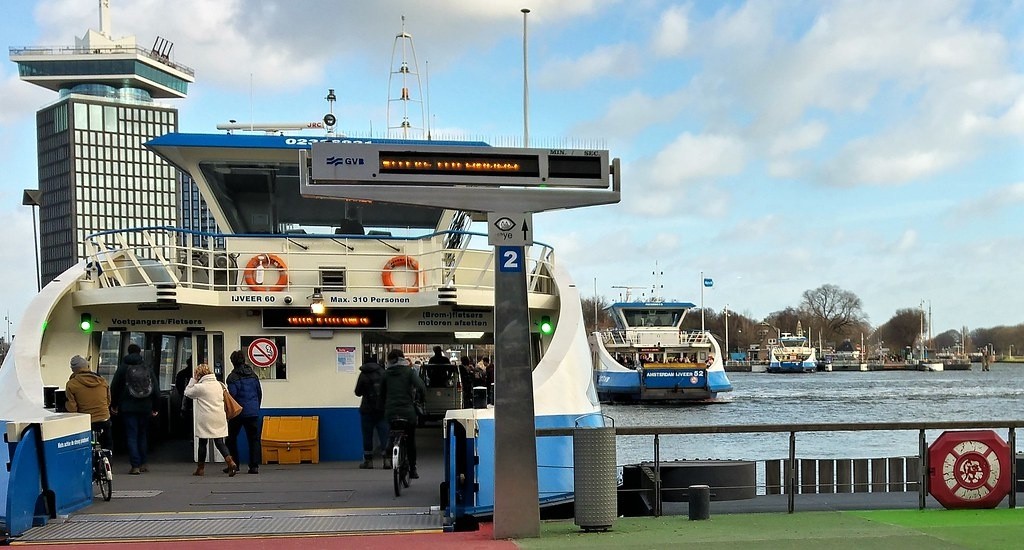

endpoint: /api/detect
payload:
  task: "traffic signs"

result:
[486,212,534,248]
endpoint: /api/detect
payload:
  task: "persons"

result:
[110,345,162,476]
[427,346,452,389]
[459,355,494,409]
[619,357,636,370]
[183,364,237,477]
[379,349,426,479]
[176,355,202,443]
[639,355,697,366]
[223,351,262,474]
[64,354,113,469]
[355,358,392,469]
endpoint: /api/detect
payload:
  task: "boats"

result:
[928,326,973,370]
[1,14,607,513]
[765,328,819,374]
[587,259,733,406]
[816,334,860,360]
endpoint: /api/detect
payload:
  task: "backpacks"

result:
[123,362,152,399]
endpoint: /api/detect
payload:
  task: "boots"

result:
[360,452,373,469]
[225,455,237,477]
[383,454,392,468]
[193,462,205,475]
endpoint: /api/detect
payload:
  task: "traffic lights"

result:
[540,312,553,336]
[80,308,93,333]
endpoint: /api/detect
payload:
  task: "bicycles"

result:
[384,413,420,497]
[88,424,114,501]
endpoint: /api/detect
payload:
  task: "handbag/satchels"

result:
[219,381,243,420]
[413,400,423,415]
[362,388,380,415]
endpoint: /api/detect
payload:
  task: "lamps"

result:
[306,287,325,315]
[80,312,91,331]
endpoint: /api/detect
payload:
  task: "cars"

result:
[419,360,462,411]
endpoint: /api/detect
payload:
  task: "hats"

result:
[71,355,89,372]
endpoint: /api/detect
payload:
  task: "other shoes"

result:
[223,465,239,472]
[409,470,419,478]
[248,467,258,474]
[128,467,140,474]
[386,441,393,455]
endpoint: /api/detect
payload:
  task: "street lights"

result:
[520,9,531,147]
[989,343,993,357]
[906,346,911,356]
[1010,344,1014,359]
[720,304,731,361]
[918,298,926,359]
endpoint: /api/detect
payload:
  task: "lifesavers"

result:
[381,255,426,293]
[244,254,288,293]
